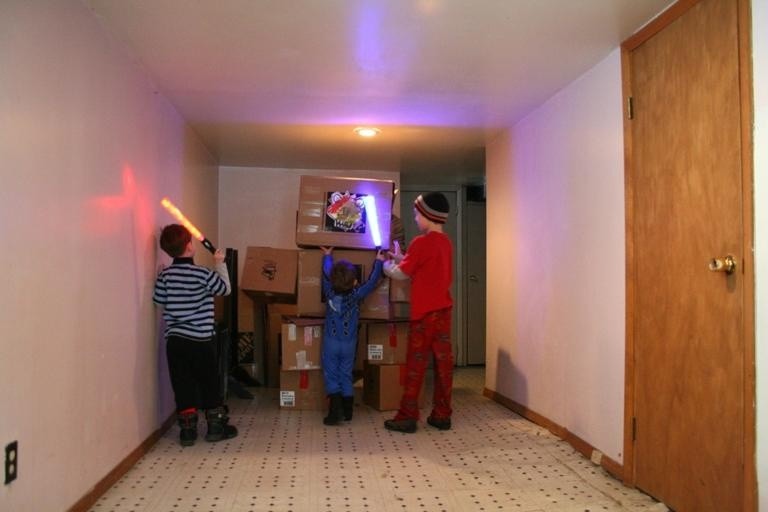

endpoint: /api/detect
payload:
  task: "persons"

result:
[152,223,238,448]
[318,245,386,425]
[382,191,455,433]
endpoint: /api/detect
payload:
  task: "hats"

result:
[412,191,449,224]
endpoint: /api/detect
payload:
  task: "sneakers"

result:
[383,418,417,434]
[426,416,452,431]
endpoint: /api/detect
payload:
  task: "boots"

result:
[176,414,199,447]
[204,413,238,442]
[323,392,355,426]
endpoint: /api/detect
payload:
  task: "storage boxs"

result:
[279,315,325,369]
[296,246,390,323]
[349,318,368,372]
[216,286,269,387]
[388,302,410,323]
[361,360,423,412]
[279,368,332,411]
[390,272,413,304]
[365,319,410,365]
[241,244,299,306]
[294,176,397,252]
